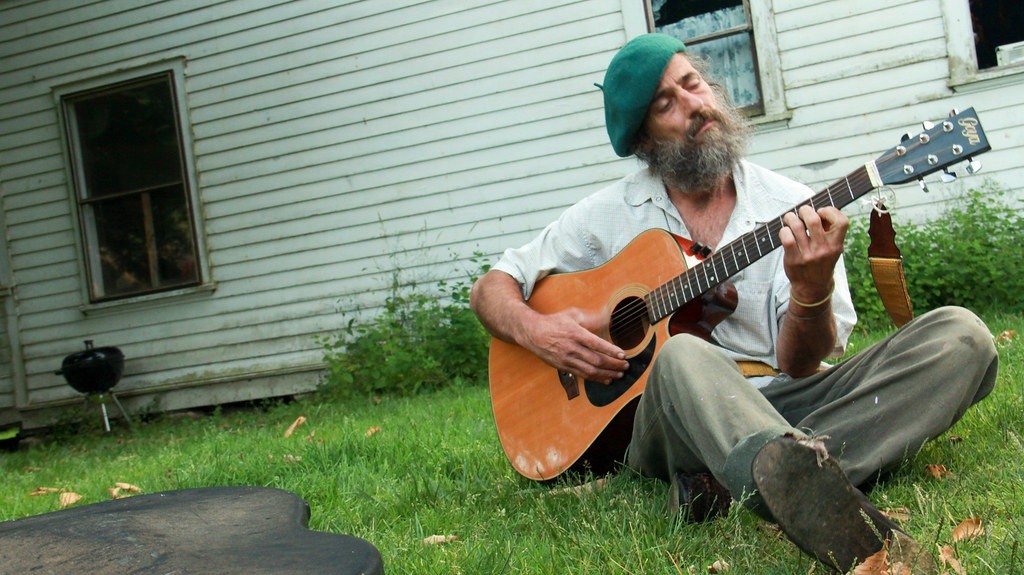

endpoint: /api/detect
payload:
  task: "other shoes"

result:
[751,438,937,575]
[671,467,730,528]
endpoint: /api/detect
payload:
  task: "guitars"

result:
[487,105,991,487]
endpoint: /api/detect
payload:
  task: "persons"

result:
[469,33,998,574]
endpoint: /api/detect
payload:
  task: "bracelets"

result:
[790,278,835,307]
[788,305,832,320]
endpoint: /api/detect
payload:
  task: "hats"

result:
[593,33,689,158]
